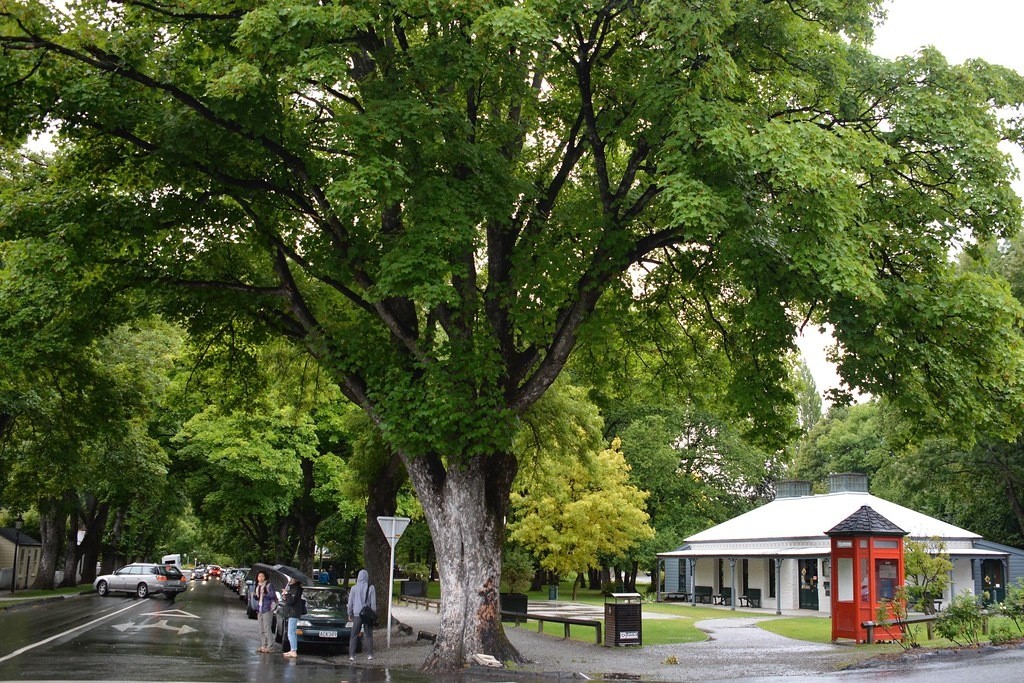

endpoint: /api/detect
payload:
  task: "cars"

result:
[190,565,364,653]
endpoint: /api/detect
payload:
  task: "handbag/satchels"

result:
[301,598,308,615]
[360,606,378,626]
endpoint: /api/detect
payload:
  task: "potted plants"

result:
[401,562,430,597]
[500,552,536,622]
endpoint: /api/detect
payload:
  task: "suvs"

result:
[93,563,187,598]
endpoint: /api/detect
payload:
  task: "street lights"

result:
[11,514,25,593]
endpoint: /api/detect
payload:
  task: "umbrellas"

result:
[244,563,289,593]
[274,564,313,595]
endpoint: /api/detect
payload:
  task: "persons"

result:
[252,571,275,653]
[319,569,338,585]
[347,570,376,660]
[282,578,303,657]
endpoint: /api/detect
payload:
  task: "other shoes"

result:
[350,656,357,661]
[367,654,378,660]
[256,647,271,653]
[283,652,298,658]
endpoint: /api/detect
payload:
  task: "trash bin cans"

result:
[604,592,642,647]
[548,588,556,600]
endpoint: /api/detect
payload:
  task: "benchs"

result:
[909,601,942,612]
[683,593,692,602]
[713,587,731,606]
[665,592,683,599]
[738,589,761,608]
[689,586,713,604]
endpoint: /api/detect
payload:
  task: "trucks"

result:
[162,554,181,569]
